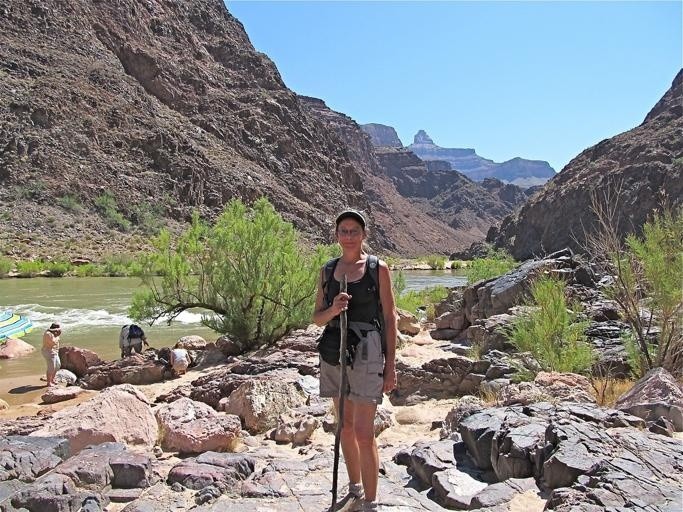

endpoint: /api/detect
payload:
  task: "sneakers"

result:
[329,491,365,511]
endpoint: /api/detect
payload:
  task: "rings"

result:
[338,307,340,313]
[393,383,397,386]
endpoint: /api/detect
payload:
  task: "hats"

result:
[48,324,60,332]
[335,211,366,227]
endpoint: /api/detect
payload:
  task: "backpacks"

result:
[127,324,143,338]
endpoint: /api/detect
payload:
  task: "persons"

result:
[311,208,398,512]
[40,323,61,388]
[119,323,150,358]
[170,341,191,376]
